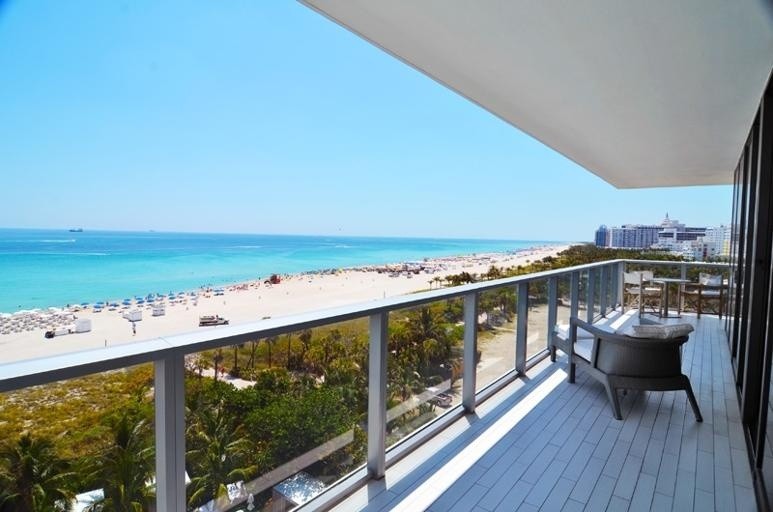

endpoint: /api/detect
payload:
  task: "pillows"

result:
[620,323,695,339]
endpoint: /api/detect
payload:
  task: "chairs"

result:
[550,317,703,422]
[621,270,724,319]
